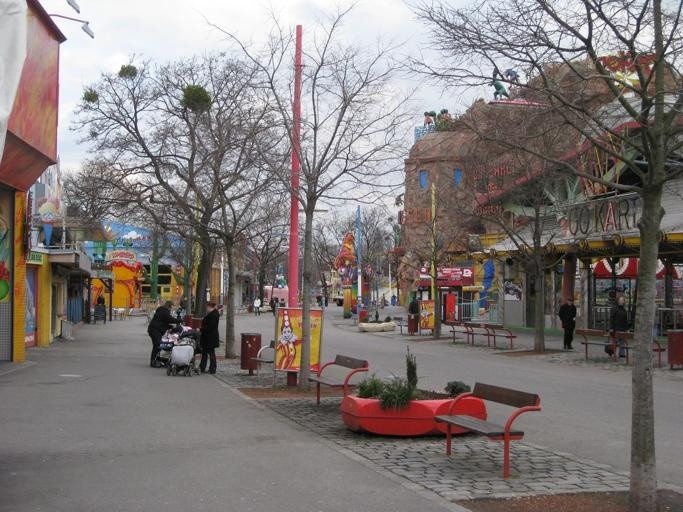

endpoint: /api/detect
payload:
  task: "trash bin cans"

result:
[408,314,418,336]
[185,315,196,326]
[666,329,683,369]
[240,333,262,375]
[190,319,204,331]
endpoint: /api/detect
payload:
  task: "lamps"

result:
[47,0,96,40]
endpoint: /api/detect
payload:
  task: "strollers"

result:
[151,321,192,367]
[167,328,201,376]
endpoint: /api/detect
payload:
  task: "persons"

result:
[558,296,577,349]
[96,293,105,305]
[194,301,221,374]
[420,303,433,328]
[278,298,286,308]
[408,296,420,314]
[608,295,629,360]
[278,309,302,371]
[269,297,276,316]
[253,296,261,317]
[147,300,184,368]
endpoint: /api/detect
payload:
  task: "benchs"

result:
[576,327,665,368]
[444,318,518,351]
[307,354,369,405]
[433,381,542,479]
[397,319,408,333]
[251,339,277,382]
